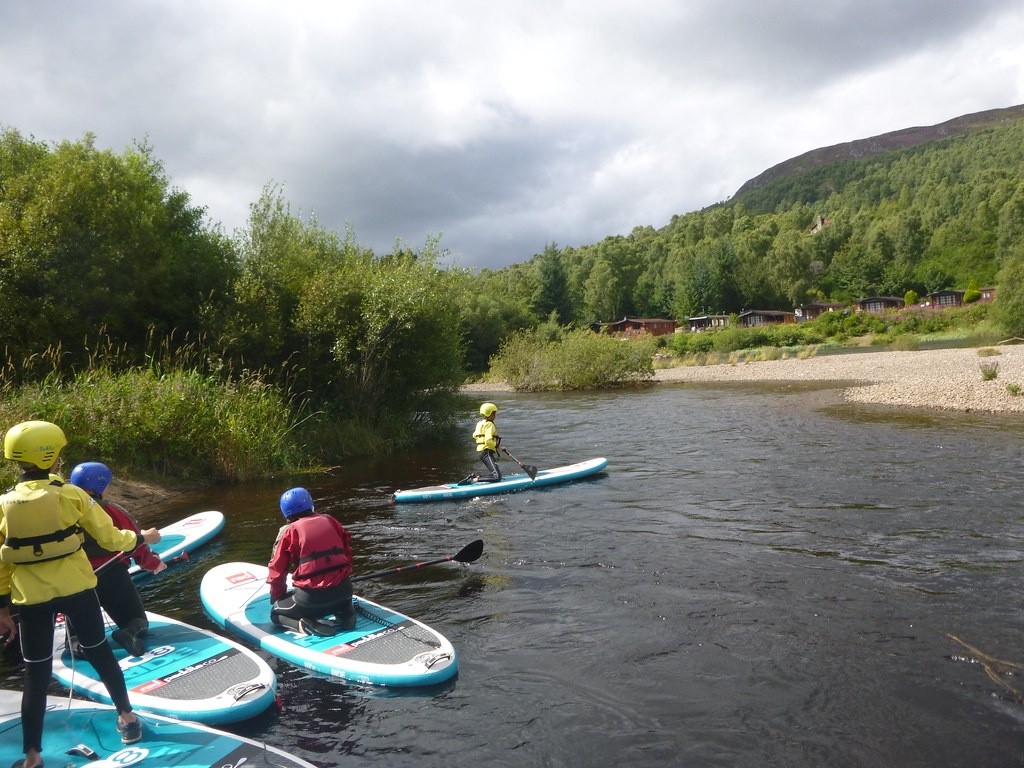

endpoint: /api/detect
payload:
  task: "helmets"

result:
[280,488,314,518]
[4,421,68,469]
[71,462,112,495]
[480,403,497,417]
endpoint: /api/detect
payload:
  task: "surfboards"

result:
[198,561,459,689]
[125,508,226,579]
[117,609,277,689]
[391,456,608,504]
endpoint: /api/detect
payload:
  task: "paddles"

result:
[10,541,146,624]
[498,445,538,482]
[283,539,484,593]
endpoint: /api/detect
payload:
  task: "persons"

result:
[0,421,168,768]
[459,403,501,486]
[265,487,356,638]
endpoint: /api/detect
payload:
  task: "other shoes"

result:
[112,630,145,656]
[457,473,476,485]
[299,618,336,637]
[11,757,44,768]
[116,715,142,743]
[342,593,356,630]
